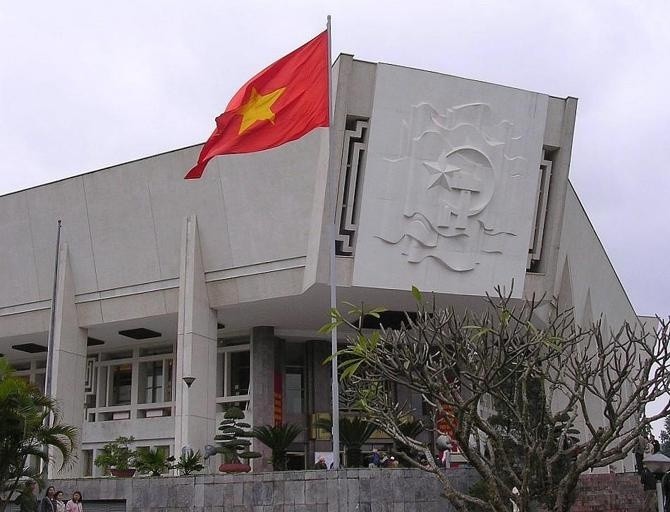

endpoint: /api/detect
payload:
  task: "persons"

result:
[649,435,660,454]
[65,491,83,512]
[631,434,647,475]
[14,480,38,512]
[314,446,453,470]
[643,436,654,473]
[54,491,65,512]
[42,486,57,512]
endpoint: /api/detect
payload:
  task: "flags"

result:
[182,29,331,179]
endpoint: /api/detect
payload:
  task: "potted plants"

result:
[138,447,169,478]
[210,408,261,474]
[93,436,142,478]
[173,452,203,477]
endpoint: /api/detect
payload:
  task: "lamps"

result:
[182,376,196,388]
